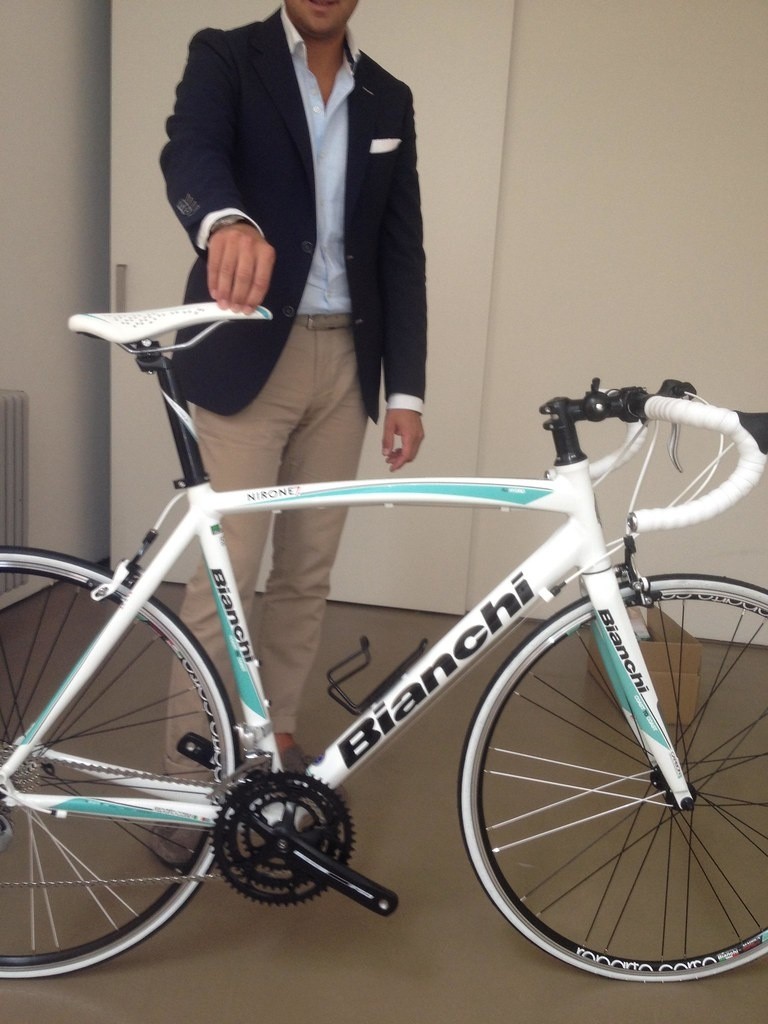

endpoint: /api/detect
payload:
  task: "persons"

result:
[144,0,428,869]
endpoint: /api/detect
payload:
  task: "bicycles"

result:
[1,299,766,983]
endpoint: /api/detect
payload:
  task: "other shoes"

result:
[277,744,346,800]
[153,813,193,865]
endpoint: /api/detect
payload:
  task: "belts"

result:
[292,312,353,330]
